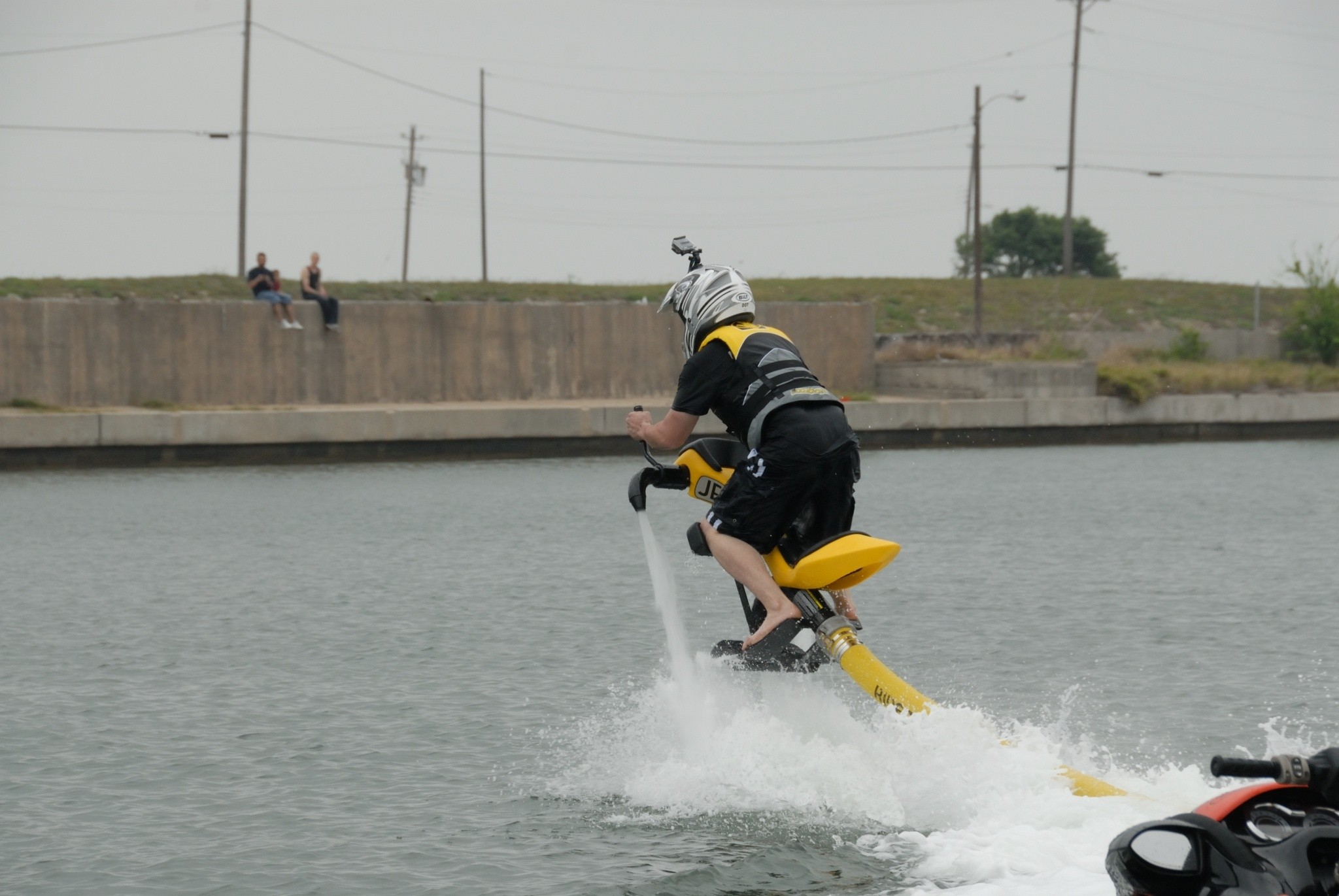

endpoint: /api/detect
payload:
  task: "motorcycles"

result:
[1105,747,1338,896]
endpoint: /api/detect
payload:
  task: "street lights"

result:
[964,86,1029,273]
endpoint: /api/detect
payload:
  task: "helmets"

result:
[656,264,756,360]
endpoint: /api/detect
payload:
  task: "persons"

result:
[300,253,339,333]
[627,266,862,649]
[249,253,303,330]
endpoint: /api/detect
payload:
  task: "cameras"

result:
[670,234,694,254]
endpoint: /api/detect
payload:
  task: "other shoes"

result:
[279,319,292,329]
[291,322,303,329]
[326,324,339,332]
